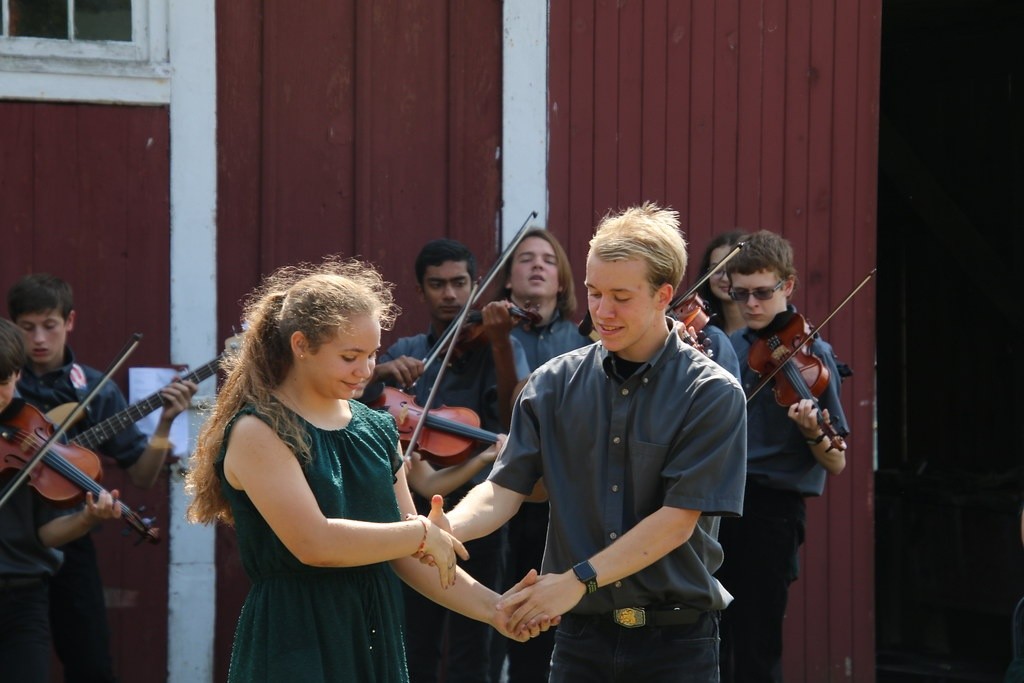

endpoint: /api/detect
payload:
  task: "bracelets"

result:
[418,518,428,555]
[799,433,826,447]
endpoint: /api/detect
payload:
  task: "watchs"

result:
[572,560,598,596]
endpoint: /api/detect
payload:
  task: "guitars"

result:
[44,327,245,470]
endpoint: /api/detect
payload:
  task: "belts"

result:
[583,607,700,630]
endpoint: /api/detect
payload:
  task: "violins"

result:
[0,398,163,543]
[365,386,507,468]
[670,288,714,358]
[744,310,848,453]
[440,298,543,371]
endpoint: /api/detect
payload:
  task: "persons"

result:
[374,227,848,683]
[184,255,562,683]
[416,199,744,683]
[0,266,198,683]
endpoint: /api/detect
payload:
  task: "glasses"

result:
[705,267,726,279]
[728,284,782,301]
